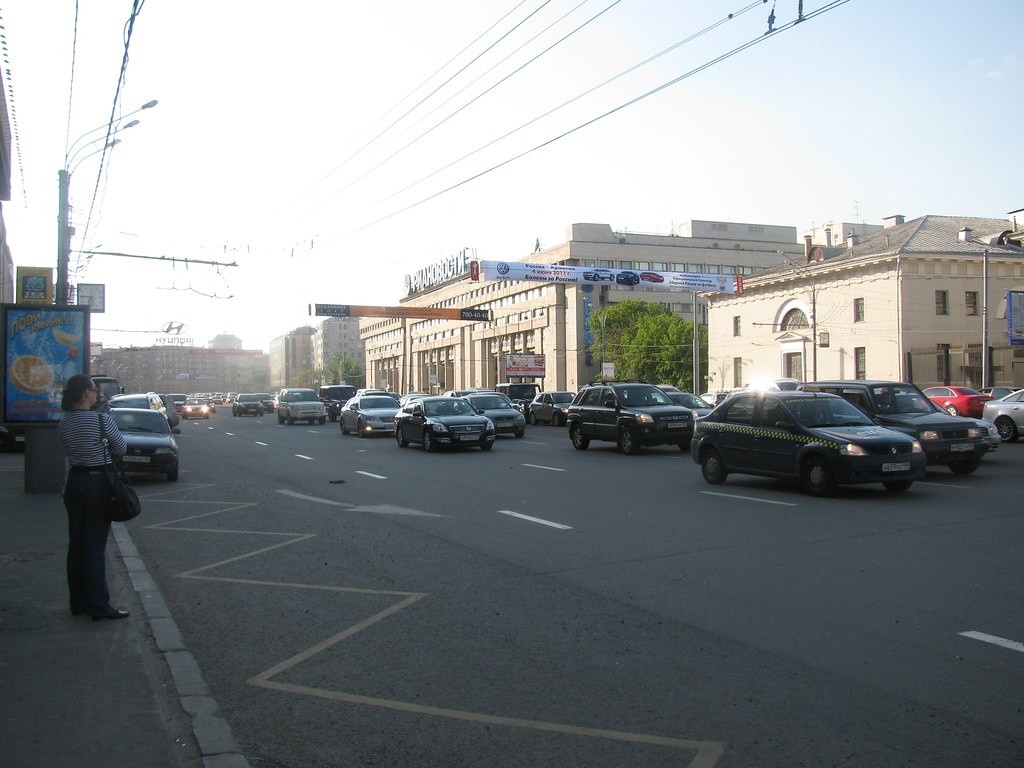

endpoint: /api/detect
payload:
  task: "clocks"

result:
[77,283,106,313]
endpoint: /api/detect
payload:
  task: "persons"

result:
[59,374,130,620]
[490,400,495,408]
[808,403,831,426]
[879,392,892,412]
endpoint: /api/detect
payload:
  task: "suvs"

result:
[566,383,698,456]
[105,392,180,430]
[277,388,326,425]
[699,378,804,404]
[495,383,542,424]
[319,385,356,422]
[795,379,1001,474]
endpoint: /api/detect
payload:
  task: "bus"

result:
[91,375,126,411]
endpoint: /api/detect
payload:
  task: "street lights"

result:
[55,99,159,304]
[777,248,817,382]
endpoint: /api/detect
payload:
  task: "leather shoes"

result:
[92,608,129,621]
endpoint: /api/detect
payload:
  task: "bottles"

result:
[49,348,80,420]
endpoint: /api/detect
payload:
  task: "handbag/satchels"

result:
[106,474,141,522]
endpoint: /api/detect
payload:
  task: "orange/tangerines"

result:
[11,355,55,394]
[52,328,81,344]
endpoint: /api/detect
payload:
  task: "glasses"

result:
[88,387,100,393]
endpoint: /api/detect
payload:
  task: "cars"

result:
[165,392,278,419]
[109,408,182,481]
[912,386,1024,443]
[691,391,927,497]
[339,388,526,451]
[665,392,713,416]
[528,391,577,426]
